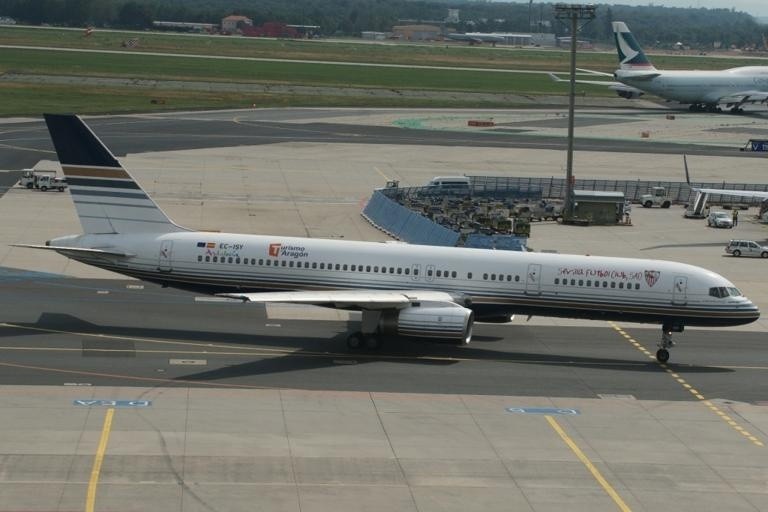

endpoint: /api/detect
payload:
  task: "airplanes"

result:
[608,18,768,113]
[437,29,508,47]
[8,112,761,364]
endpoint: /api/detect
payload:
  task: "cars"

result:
[726,239,768,258]
[708,211,734,229]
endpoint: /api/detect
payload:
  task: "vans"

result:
[418,176,471,198]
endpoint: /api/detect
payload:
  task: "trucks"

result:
[20,169,68,192]
